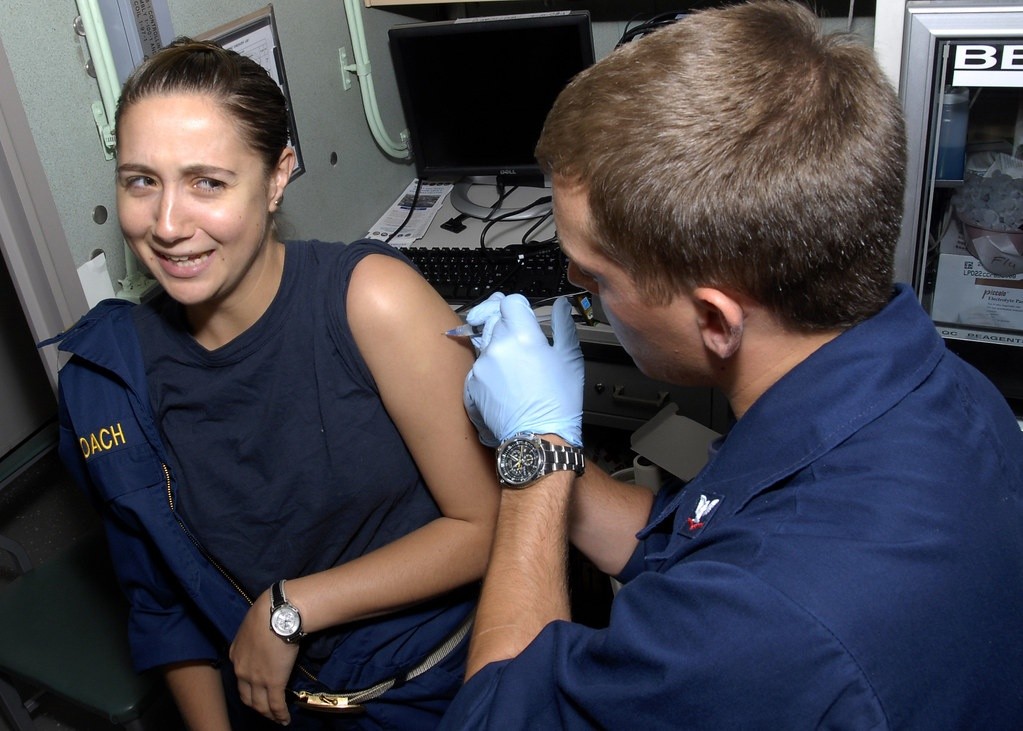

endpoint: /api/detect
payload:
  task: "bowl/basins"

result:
[955,211,1023,260]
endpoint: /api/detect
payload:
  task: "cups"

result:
[927,87,970,180]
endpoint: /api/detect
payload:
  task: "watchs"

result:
[494,433,586,491]
[270,579,310,645]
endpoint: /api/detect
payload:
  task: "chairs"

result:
[0,417,186,730]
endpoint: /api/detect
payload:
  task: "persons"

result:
[55,37,500,730]
[447,0,1023,730]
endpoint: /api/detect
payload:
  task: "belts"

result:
[293,601,480,709]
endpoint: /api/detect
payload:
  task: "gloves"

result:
[462,293,584,448]
[463,291,505,446]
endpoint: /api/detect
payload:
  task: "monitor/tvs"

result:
[387,10,595,222]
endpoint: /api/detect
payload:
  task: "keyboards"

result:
[392,244,592,307]
